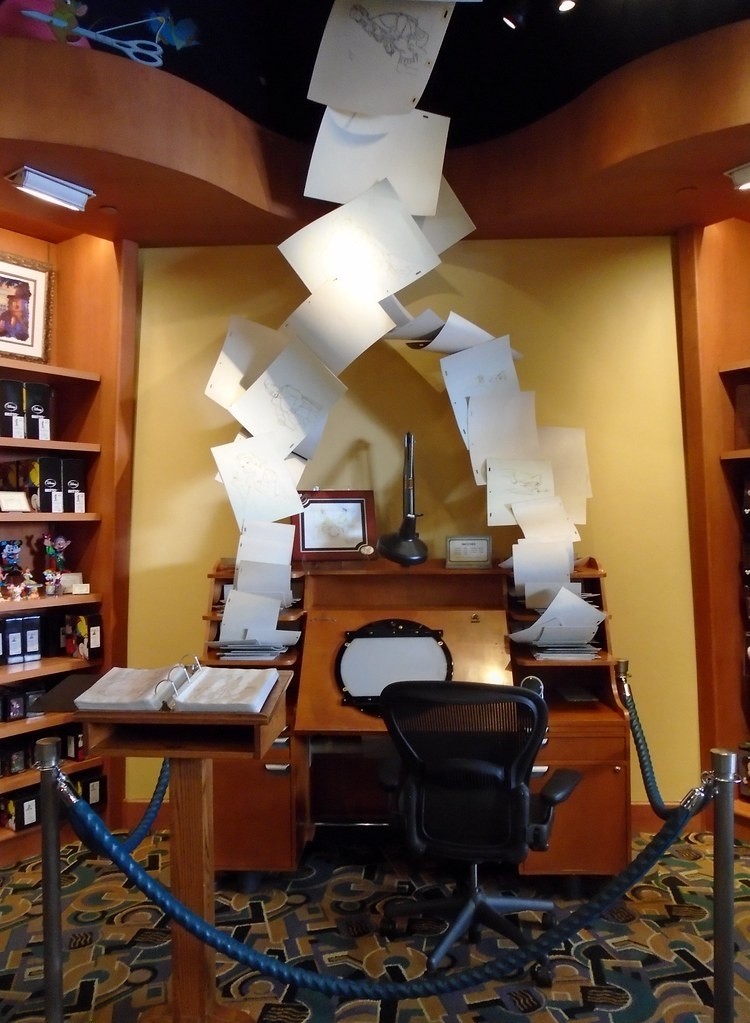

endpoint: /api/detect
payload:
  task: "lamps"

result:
[3,165,96,212]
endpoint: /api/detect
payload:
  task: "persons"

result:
[23,567,36,584]
[44,536,71,574]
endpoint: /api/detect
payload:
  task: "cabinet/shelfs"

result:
[0,356,106,867]
[717,360,750,844]
[198,556,633,898]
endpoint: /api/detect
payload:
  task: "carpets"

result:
[0,831,750,1023]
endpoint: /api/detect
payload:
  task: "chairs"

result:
[378,681,584,972]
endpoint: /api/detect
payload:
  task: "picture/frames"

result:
[290,490,379,562]
[445,535,493,569]
[0,250,58,366]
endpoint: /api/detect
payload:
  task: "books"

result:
[75,655,280,715]
